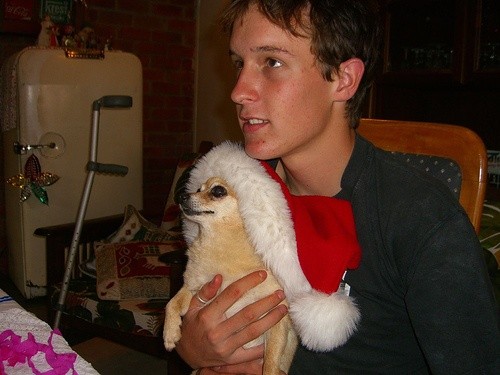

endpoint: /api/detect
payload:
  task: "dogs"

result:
[163,175,300,375]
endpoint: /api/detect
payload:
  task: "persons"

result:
[174,0,500,375]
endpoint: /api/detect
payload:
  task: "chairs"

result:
[33,141,215,375]
[355,117,488,235]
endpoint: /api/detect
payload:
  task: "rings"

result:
[196,293,208,305]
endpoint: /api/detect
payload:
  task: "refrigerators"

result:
[3,48,144,299]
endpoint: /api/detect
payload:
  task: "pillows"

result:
[160,158,203,236]
[78,204,178,279]
[93,240,188,298]
